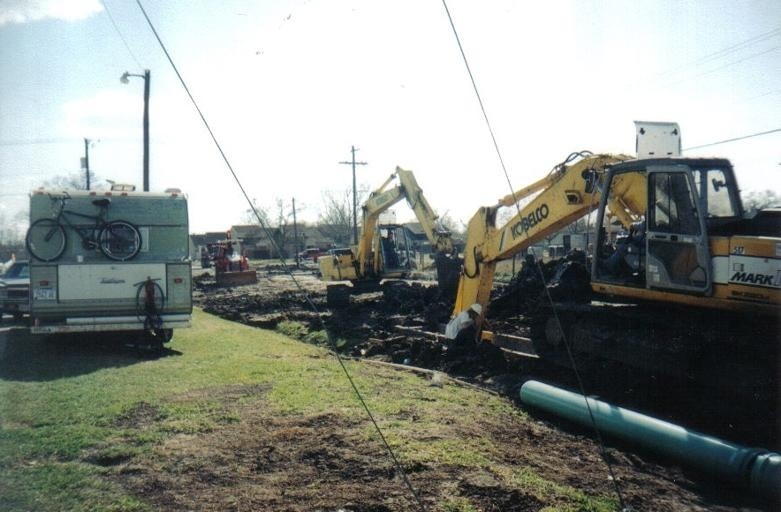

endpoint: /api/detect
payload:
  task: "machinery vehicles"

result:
[215,229,256,283]
[443,119,781,388]
[315,164,463,297]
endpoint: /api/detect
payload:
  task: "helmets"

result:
[470,303,482,318]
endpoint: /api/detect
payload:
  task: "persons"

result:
[602,208,658,275]
[386,232,399,266]
[445,303,483,364]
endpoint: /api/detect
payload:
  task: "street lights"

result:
[120,66,150,192]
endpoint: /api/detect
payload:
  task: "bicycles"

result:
[26,192,141,260]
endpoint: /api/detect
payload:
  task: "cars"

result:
[298,248,319,259]
[0,260,30,321]
